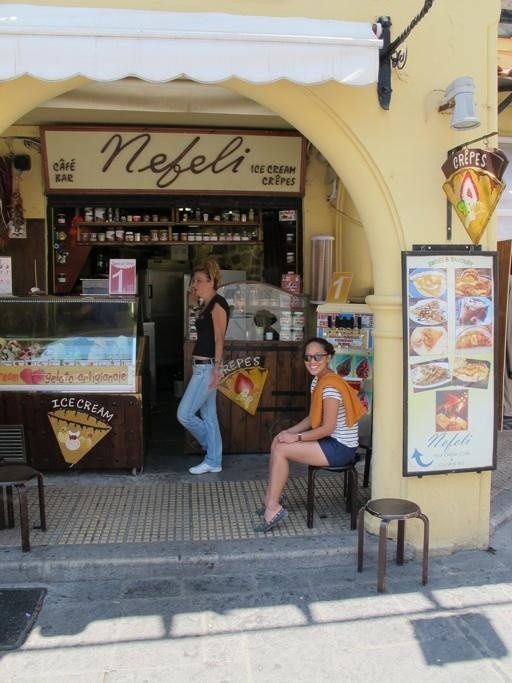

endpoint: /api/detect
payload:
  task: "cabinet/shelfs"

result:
[0,295,149,473]
[76,199,264,248]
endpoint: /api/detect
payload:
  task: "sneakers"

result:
[254,506,288,532]
[189,461,222,474]
[256,495,288,515]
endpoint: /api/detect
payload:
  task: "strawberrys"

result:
[32,371,43,384]
[20,368,32,384]
[234,374,254,393]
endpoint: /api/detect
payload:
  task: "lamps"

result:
[431,77,481,134]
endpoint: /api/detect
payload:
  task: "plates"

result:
[409,271,489,389]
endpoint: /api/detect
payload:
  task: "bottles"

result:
[210,230,259,241]
[279,310,303,341]
[248,208,254,220]
[201,210,209,221]
[265,327,274,340]
[108,207,119,222]
[57,213,66,223]
[82,226,169,242]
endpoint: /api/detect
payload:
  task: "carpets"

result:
[0,586,49,650]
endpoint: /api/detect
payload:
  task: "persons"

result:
[176,257,230,474]
[254,336,368,531]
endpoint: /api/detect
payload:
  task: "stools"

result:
[0,461,45,554]
[355,500,432,593]
[304,453,363,529]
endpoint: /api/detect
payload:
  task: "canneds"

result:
[286,252,295,264]
[285,233,294,242]
[57,273,67,283]
[84,207,93,222]
[58,214,66,224]
[80,226,168,242]
[279,311,305,342]
[172,232,241,242]
[94,208,106,222]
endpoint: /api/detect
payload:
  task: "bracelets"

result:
[215,360,221,364]
[298,432,302,441]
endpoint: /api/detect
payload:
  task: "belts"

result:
[195,360,214,364]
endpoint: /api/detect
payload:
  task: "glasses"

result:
[303,354,328,362]
